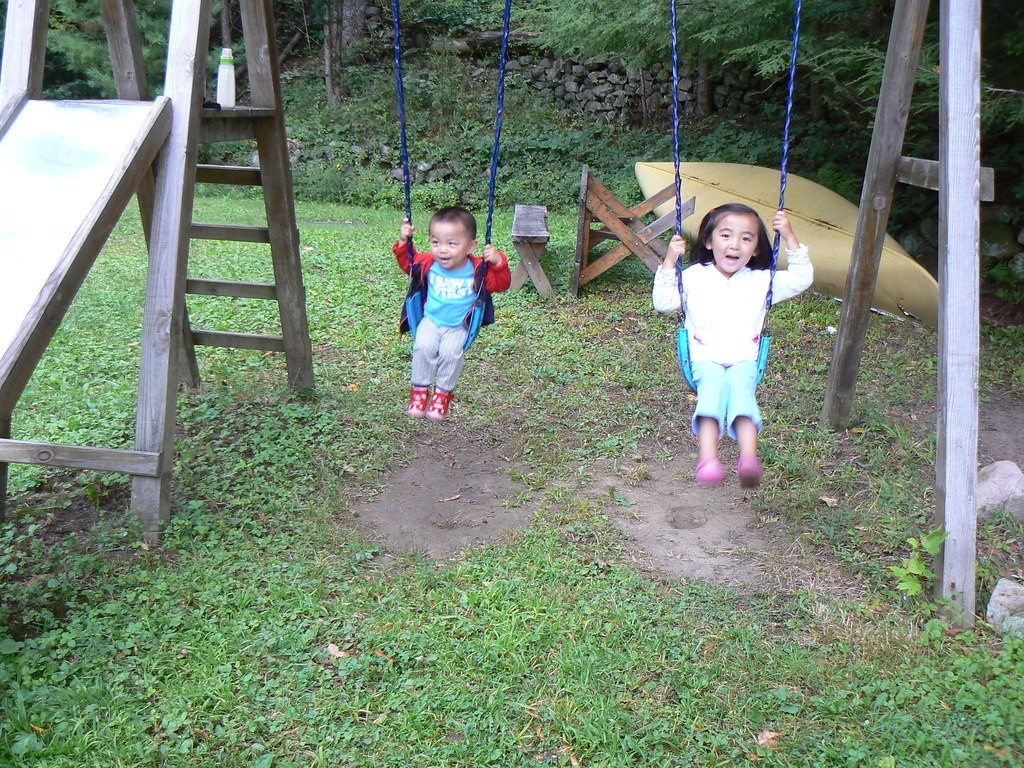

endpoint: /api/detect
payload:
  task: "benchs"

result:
[509,205,554,298]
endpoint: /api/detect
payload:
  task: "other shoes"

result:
[696,456,722,481]
[738,455,760,488]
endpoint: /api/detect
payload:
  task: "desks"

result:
[572,163,696,296]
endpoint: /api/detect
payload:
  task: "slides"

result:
[1,88,171,420]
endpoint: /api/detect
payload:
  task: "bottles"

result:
[217,48,236,108]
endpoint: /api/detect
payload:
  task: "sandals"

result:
[426,391,454,420]
[407,387,430,418]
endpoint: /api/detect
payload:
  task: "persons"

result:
[653,203,814,488]
[392,207,511,421]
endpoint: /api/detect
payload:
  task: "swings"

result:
[388,1,514,355]
[665,0,803,399]
